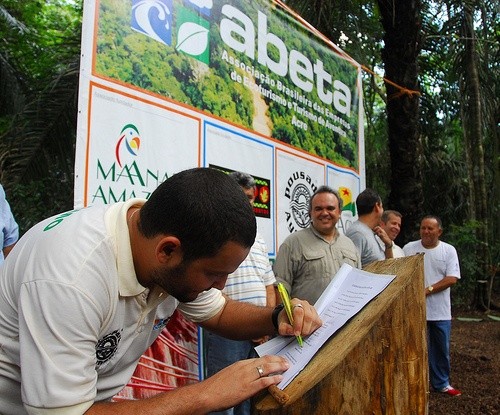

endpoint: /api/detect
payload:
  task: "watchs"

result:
[428,286,433,294]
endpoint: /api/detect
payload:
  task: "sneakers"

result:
[439,384,462,398]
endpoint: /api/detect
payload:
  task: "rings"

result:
[256,366,264,378]
[291,304,304,311]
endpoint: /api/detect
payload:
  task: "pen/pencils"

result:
[275,280,305,349]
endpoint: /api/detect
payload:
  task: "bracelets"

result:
[271,303,286,335]
[385,241,393,248]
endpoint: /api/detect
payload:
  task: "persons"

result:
[0,168,323,415]
[345,188,393,269]
[375,210,406,258]
[203,171,276,415]
[403,215,462,397]
[272,185,362,306]
